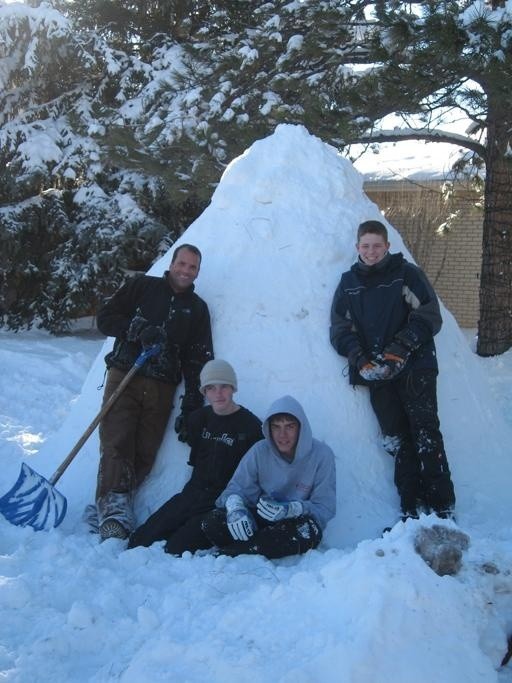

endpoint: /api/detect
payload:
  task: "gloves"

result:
[226,494,258,541]
[255,497,303,522]
[355,340,412,381]
[175,386,204,445]
[124,316,167,350]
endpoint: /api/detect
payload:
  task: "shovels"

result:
[0,343,160,530]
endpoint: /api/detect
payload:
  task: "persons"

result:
[125,360,264,554]
[84,245,215,540]
[329,220,455,530]
[200,395,336,555]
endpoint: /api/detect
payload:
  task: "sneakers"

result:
[100,518,128,541]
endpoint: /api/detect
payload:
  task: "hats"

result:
[198,359,238,395]
